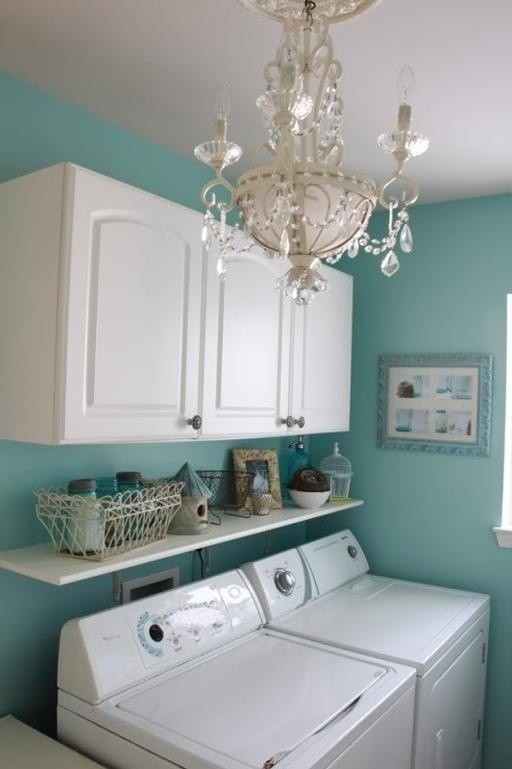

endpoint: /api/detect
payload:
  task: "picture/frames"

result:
[231,447,285,513]
[374,352,493,460]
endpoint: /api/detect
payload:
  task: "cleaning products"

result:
[286,435,311,500]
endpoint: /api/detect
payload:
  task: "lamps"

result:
[194,1,429,305]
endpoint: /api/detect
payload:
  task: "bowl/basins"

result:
[287,488,331,509]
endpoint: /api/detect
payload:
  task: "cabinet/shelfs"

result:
[1,162,204,449]
[203,215,356,445]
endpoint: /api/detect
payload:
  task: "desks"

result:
[1,494,371,607]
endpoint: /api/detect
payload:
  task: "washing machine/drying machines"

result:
[239,527,491,769]
[57,568,417,768]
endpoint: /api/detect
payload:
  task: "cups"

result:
[252,491,273,515]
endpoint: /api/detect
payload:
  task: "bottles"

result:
[116,470,145,540]
[97,476,122,545]
[66,478,104,554]
